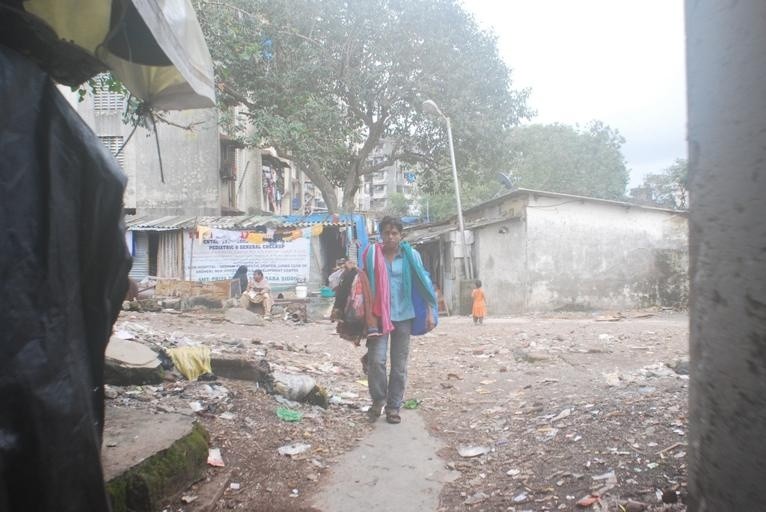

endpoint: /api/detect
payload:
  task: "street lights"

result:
[422,100,470,280]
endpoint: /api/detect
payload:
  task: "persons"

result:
[471,280,488,325]
[233,265,273,317]
[325,256,357,293]
[360,215,436,426]
[430,280,443,313]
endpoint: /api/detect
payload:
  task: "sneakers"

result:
[366,397,386,419]
[383,405,401,425]
